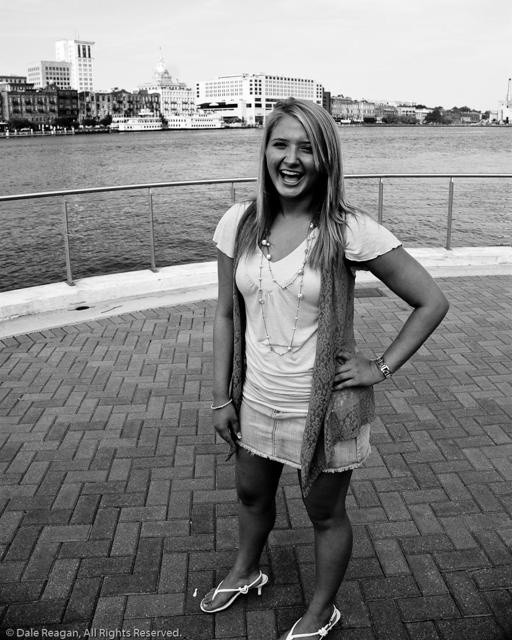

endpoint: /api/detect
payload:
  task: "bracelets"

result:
[210,398,233,411]
[373,356,393,382]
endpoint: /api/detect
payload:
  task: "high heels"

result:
[199,570,269,613]
[285,604,341,640]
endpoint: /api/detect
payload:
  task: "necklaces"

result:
[258,220,315,356]
[266,230,317,290]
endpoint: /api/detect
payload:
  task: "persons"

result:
[200,96,452,640]
